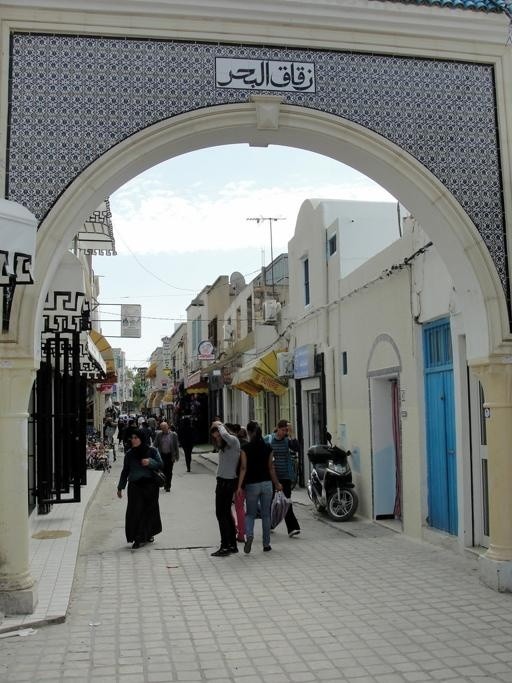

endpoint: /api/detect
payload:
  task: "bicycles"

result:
[290,452,299,491]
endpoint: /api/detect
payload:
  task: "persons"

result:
[117,429,164,549]
[103,407,195,492]
[211,416,300,556]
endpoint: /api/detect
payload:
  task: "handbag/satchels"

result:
[153,470,166,488]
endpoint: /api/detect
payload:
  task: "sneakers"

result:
[212,524,300,557]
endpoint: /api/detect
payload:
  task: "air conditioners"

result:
[277,352,294,378]
[223,325,232,340]
[262,299,277,321]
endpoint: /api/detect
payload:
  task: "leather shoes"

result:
[148,535,154,542]
[132,541,140,549]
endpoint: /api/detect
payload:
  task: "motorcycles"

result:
[305,429,359,521]
